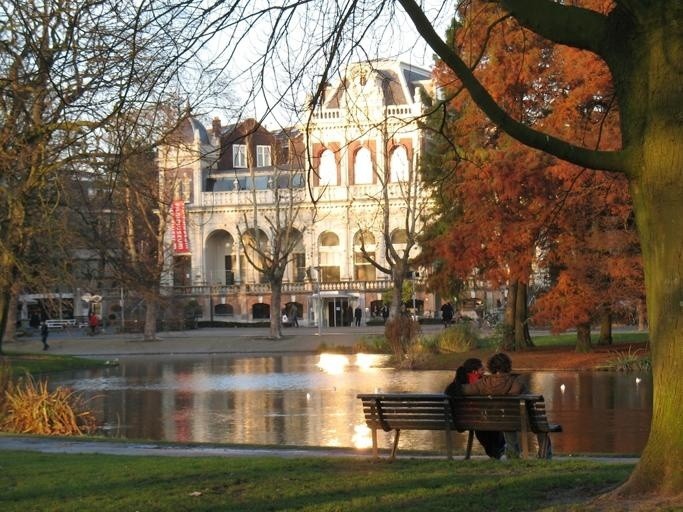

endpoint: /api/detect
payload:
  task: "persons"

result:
[444,352,539,459]
[347,303,353,326]
[289,304,299,328]
[354,303,363,327]
[379,303,389,321]
[89,312,97,332]
[440,300,454,328]
[444,357,506,461]
[31,311,39,328]
[38,319,50,350]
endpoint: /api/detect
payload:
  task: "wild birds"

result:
[635,376,641,384]
[560,383,566,391]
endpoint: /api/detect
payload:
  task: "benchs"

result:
[357,392,567,464]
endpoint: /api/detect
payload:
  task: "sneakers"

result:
[496,451,506,461]
